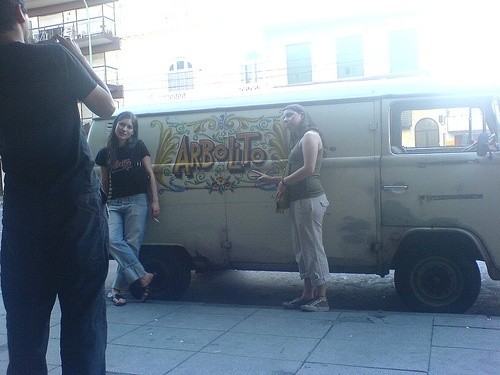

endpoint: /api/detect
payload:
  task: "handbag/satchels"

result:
[133,140,149,192]
[275,160,292,214]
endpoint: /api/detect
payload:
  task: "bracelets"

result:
[281,178,286,186]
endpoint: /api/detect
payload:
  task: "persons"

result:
[94,112,161,306]
[251,104,331,311]
[0,0,115,375]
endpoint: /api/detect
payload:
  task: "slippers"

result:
[111,294,127,306]
[141,271,158,303]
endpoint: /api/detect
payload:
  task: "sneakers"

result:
[282,297,313,309]
[301,298,329,311]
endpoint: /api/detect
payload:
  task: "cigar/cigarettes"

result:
[154,217,159,223]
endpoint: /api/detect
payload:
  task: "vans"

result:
[88,76,499,311]
